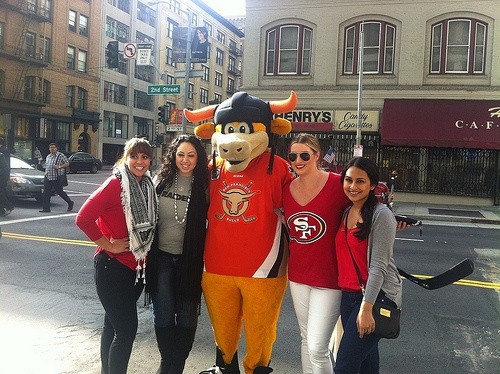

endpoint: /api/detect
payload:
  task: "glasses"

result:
[289,153,314,162]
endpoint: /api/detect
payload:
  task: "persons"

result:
[0,136,14,217]
[145,134,209,374]
[334,157,401,374]
[75,139,158,374]
[39,143,74,212]
[281,133,410,374]
[34,146,41,166]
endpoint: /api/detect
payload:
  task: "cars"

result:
[5,154,57,202]
[37,151,102,174]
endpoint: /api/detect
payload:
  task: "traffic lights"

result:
[106,40,119,68]
[156,134,164,144]
[157,105,169,125]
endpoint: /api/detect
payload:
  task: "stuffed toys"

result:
[184,90,298,374]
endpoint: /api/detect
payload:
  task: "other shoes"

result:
[39,208,51,212]
[3,208,12,216]
[68,201,74,211]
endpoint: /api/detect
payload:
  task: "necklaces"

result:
[174,169,194,224]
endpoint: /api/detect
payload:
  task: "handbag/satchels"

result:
[57,175,68,186]
[363,289,401,340]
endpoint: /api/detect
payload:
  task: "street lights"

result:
[146,0,193,133]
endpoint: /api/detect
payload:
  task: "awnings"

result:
[381,99,500,150]
